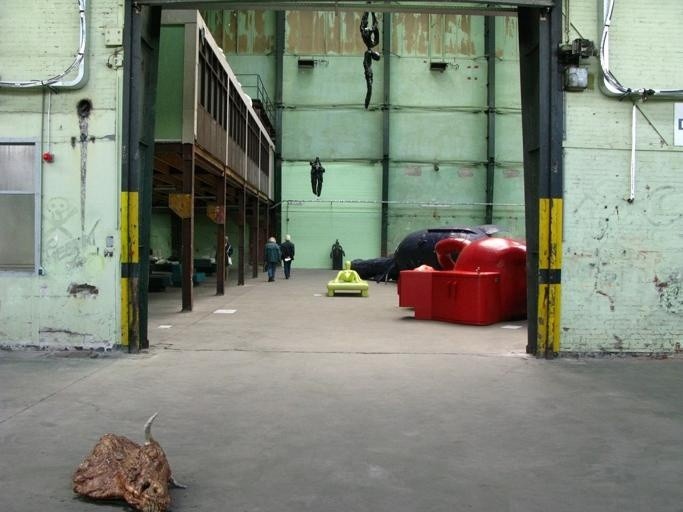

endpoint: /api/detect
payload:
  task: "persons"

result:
[214,236,233,281]
[262,236,282,282]
[278,234,294,279]
[333,260,362,283]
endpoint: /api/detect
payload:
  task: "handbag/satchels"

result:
[228,257,232,265]
[263,264,268,272]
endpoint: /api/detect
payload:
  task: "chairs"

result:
[150,256,217,292]
